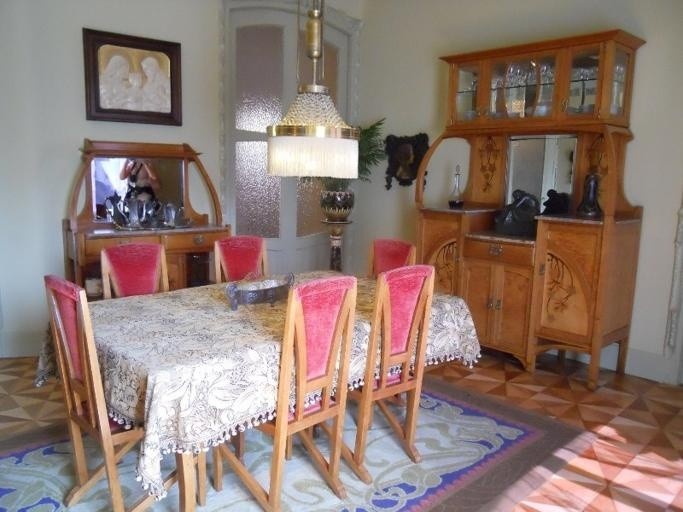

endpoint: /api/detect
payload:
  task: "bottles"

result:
[447,164,463,210]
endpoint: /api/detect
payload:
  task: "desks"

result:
[32,265,483,512]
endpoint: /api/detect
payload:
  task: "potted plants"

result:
[299,115,392,224]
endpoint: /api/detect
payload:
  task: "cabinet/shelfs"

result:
[55,137,230,300]
[412,25,645,391]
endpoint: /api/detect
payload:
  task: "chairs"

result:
[342,264,438,485]
[364,237,419,279]
[203,234,268,285]
[44,270,211,512]
[92,239,170,297]
[209,268,360,511]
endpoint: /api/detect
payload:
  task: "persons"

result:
[493,189,539,222]
[120,158,163,225]
[541,188,571,215]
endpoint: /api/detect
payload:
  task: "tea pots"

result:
[103,190,120,224]
[115,189,191,230]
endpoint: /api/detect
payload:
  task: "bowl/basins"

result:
[228,279,290,310]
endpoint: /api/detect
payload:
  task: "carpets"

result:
[0,366,600,512]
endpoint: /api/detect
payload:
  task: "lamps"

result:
[264,0,361,184]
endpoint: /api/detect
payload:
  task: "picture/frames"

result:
[78,24,184,132]
